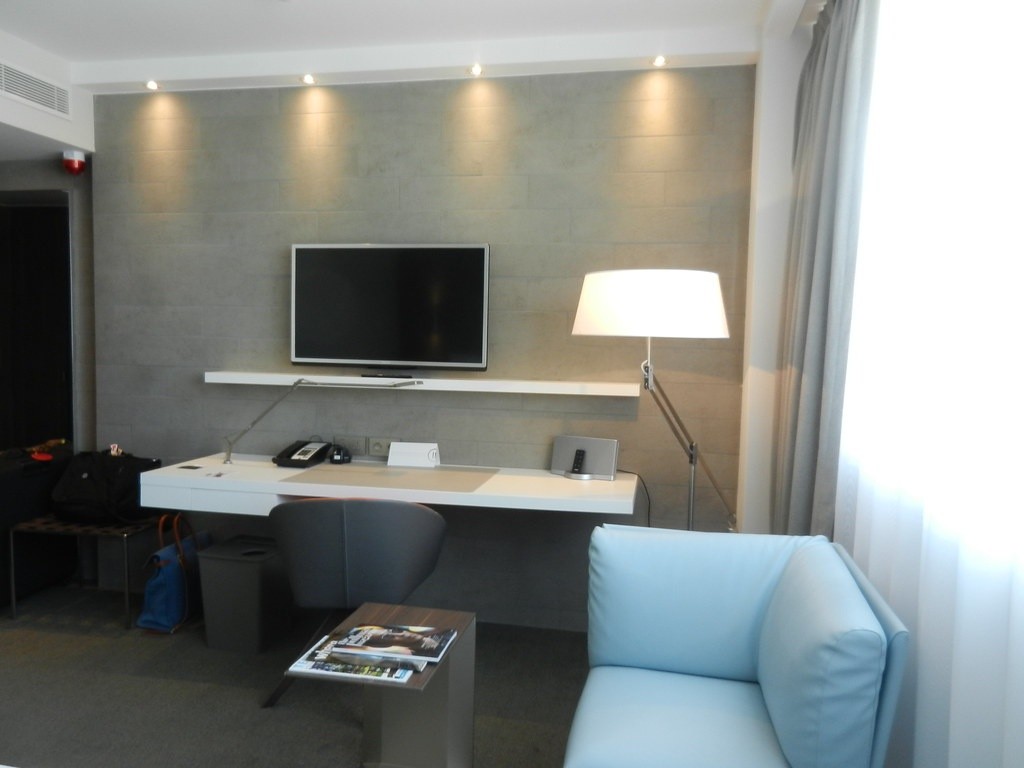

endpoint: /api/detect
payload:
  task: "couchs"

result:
[563,524,909,768]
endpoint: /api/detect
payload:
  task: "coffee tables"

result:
[285,601,475,768]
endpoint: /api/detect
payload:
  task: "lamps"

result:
[571,268,735,531]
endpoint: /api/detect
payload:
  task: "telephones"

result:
[272,440,332,468]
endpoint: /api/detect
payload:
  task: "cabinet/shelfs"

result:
[140,371,641,516]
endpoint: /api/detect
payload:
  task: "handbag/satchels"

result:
[135,514,209,634]
[52,449,161,525]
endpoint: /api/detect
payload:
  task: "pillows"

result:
[757,535,888,768]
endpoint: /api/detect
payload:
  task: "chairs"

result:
[267,499,447,707]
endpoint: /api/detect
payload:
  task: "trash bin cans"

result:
[196,534,283,657]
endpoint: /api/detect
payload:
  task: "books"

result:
[289,618,458,684]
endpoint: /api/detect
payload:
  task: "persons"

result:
[335,627,455,657]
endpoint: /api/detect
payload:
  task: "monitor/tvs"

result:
[289,242,490,372]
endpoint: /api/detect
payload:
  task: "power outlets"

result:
[368,437,400,456]
[333,435,366,455]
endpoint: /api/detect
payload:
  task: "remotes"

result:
[572,449,585,473]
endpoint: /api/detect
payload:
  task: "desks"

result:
[9,516,157,629]
